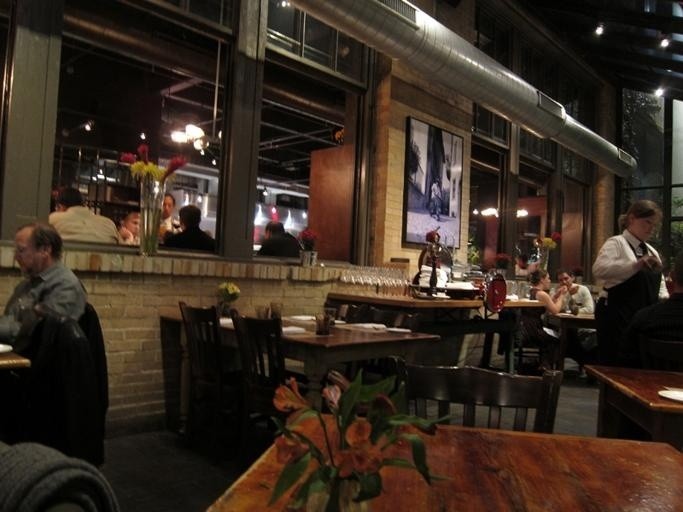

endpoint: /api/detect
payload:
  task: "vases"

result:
[537,248,547,272]
[138,179,164,255]
[575,273,582,283]
[496,268,507,280]
[299,251,317,266]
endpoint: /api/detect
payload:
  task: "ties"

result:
[639,242,648,256]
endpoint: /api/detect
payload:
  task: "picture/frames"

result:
[399,115,464,250]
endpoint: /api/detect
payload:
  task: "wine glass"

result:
[335,263,410,299]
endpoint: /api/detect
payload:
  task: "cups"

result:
[269,302,283,321]
[314,313,331,336]
[255,304,270,321]
[321,307,336,326]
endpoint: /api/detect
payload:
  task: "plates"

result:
[658,390,683,402]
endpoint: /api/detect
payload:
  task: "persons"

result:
[254,220,301,259]
[588,199,669,368]
[514,254,540,278]
[46,186,120,245]
[110,204,141,244]
[154,205,218,255]
[612,249,681,374]
[548,266,595,379]
[509,268,567,373]
[157,192,181,237]
[486,253,516,281]
[0,221,89,404]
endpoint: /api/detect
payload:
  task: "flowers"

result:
[296,229,321,250]
[118,145,187,253]
[494,253,512,269]
[573,266,582,275]
[215,280,240,300]
[532,231,562,267]
